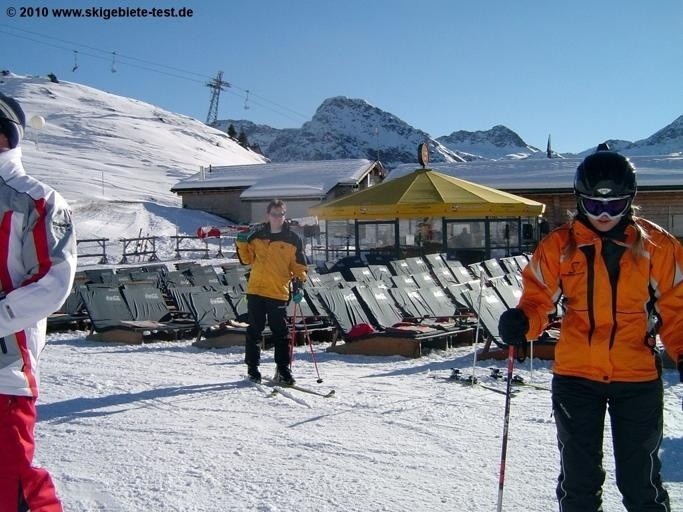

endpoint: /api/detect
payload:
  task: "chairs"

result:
[53,248,564,359]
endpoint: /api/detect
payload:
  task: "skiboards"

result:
[429,367,552,398]
[240,373,336,398]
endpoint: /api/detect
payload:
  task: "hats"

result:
[0,91,25,149]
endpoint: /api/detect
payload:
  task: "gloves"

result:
[238,233,248,241]
[293,293,302,303]
[498,308,529,345]
[677,361,683,383]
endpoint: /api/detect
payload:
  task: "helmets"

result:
[574,150,637,220]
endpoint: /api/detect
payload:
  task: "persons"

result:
[235,199,309,386]
[47,72,59,82]
[0,93,77,512]
[497,151,683,511]
[458,225,484,248]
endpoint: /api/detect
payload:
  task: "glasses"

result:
[574,190,632,220]
[271,210,285,217]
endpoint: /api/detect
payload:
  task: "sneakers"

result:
[248,365,296,385]
